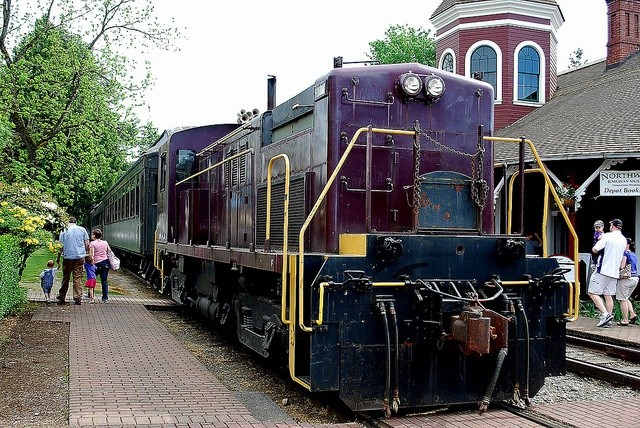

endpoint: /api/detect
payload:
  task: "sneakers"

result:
[103,299,109,303]
[596,314,614,326]
[88,301,93,303]
[46,302,50,305]
[602,322,611,327]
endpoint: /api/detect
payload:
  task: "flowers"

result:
[555,181,578,199]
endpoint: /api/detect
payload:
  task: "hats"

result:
[593,220,604,227]
[609,219,622,229]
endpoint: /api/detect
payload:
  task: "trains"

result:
[79,57,581,413]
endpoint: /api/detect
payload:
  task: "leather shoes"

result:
[75,301,81,305]
[57,301,66,305]
[617,321,629,326]
[628,315,638,324]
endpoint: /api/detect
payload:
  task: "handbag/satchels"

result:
[107,251,120,270]
[619,264,631,279]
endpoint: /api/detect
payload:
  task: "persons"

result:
[84,255,97,302]
[589,222,604,267]
[89,229,118,301]
[617,239,639,329]
[38,256,58,298]
[589,220,627,325]
[525,232,542,255]
[58,215,89,303]
